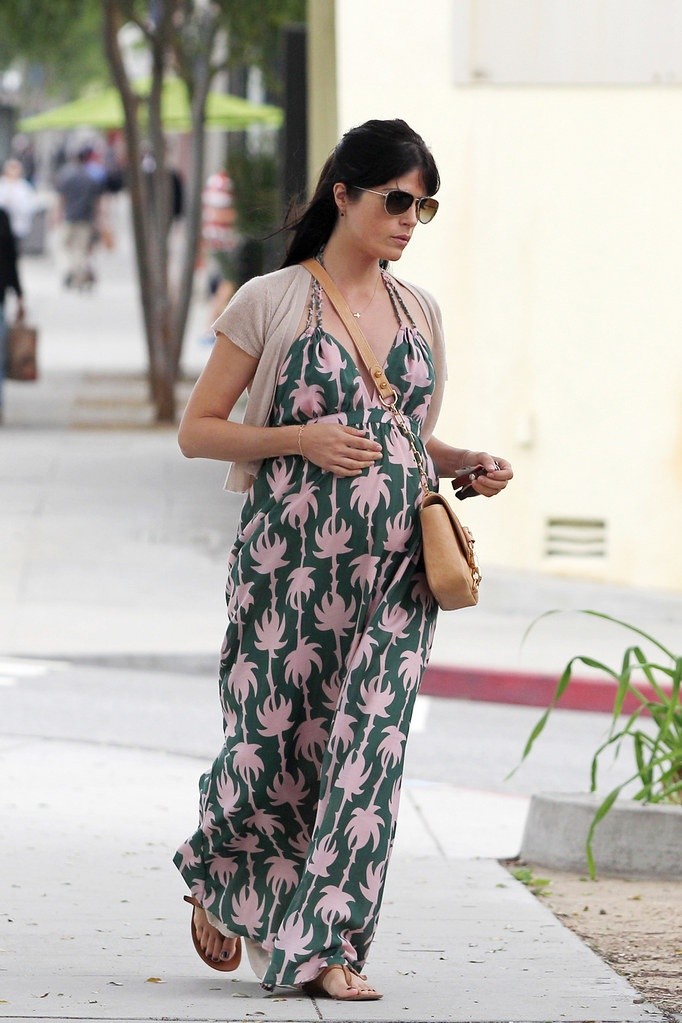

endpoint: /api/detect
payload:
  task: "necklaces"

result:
[353,276,381,318]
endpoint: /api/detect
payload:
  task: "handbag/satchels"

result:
[7,299,36,380]
[419,493,482,611]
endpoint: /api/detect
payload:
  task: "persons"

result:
[0,158,36,241]
[97,152,124,250]
[143,151,185,286]
[51,141,107,286]
[202,175,244,296]
[0,210,26,378]
[177,117,514,1001]
[225,151,295,395]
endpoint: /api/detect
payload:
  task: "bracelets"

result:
[298,424,308,461]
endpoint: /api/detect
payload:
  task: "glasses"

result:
[354,186,439,224]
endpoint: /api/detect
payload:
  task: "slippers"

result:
[302,963,383,1000]
[183,895,242,972]
